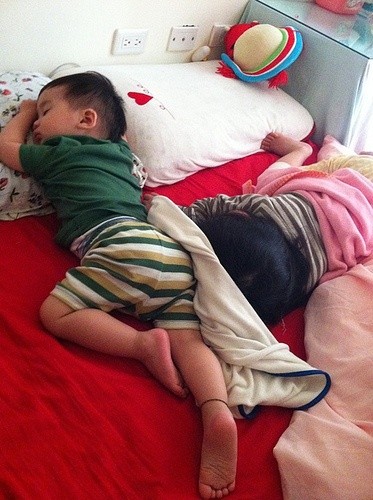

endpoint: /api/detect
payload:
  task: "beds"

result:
[0,59,373,500]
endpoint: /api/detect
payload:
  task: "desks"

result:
[239,0,373,155]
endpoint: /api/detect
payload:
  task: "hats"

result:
[221,24,303,83]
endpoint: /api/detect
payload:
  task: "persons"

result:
[0,70,238,500]
[142,131,373,329]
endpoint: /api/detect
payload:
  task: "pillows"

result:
[51,61,314,188]
[0,71,80,222]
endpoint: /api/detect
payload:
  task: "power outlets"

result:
[111,28,149,56]
[166,28,199,51]
[209,26,235,44]
